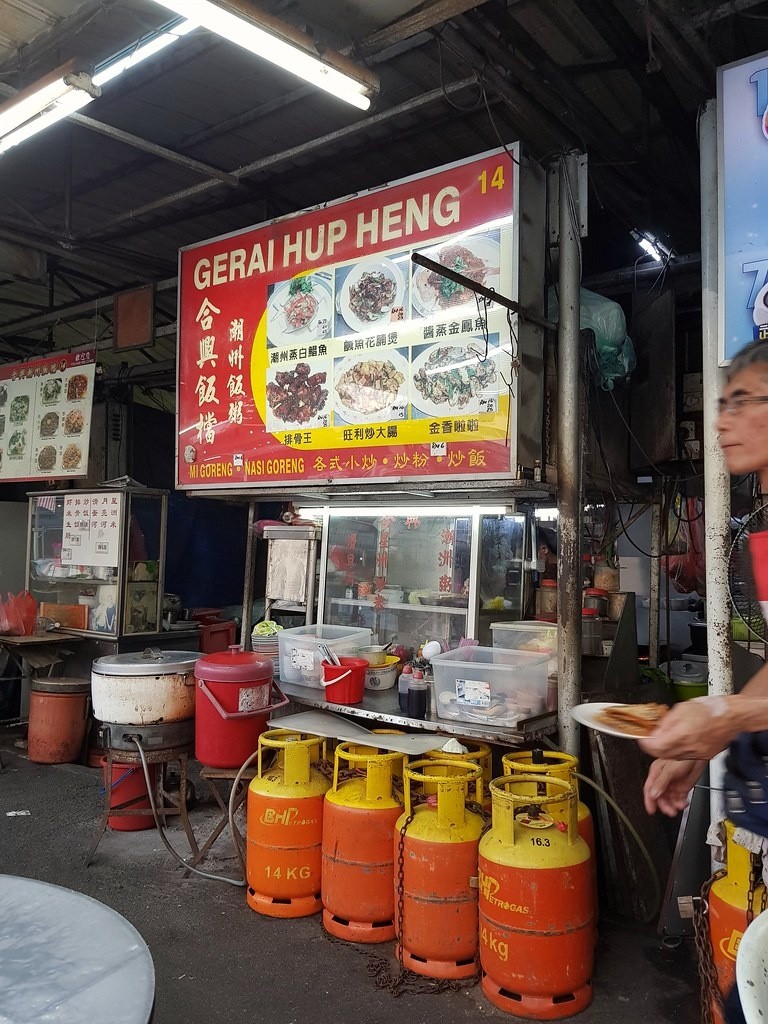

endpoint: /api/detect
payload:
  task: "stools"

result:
[83,744,203,869]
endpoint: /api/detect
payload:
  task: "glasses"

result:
[717,394,768,415]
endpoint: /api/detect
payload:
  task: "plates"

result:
[570,702,655,739]
[0,374,90,473]
[369,655,401,668]
[251,635,280,676]
[265,236,500,432]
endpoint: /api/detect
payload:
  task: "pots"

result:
[687,617,708,651]
[90,646,208,725]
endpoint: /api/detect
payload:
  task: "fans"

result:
[727,502,768,644]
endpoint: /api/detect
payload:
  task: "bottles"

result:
[406,670,426,719]
[36,616,61,631]
[584,588,610,621]
[580,608,603,656]
[344,583,358,599]
[540,579,557,618]
[593,556,620,592]
[397,665,414,713]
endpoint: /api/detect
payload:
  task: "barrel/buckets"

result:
[193,645,290,769]
[364,662,397,691]
[98,754,156,831]
[320,657,369,705]
[27,677,91,764]
[673,681,708,703]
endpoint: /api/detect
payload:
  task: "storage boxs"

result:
[491,621,558,676]
[278,623,373,690]
[430,645,551,727]
[193,614,237,654]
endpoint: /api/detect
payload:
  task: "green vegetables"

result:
[443,364,476,400]
[289,275,313,296]
[439,256,467,299]
[639,665,668,681]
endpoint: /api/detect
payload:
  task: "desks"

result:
[0,633,84,769]
[270,675,559,753]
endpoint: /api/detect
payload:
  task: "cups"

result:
[34,617,47,637]
[358,581,371,600]
[372,576,385,595]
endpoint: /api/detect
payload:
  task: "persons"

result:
[514,526,557,587]
[638,337,768,1024]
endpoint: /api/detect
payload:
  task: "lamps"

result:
[152,0,381,115]
[0,56,102,156]
[630,228,676,261]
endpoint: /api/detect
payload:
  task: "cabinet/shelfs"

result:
[583,592,640,692]
[263,525,366,627]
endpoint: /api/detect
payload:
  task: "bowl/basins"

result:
[374,589,404,603]
[358,645,386,664]
[418,596,442,606]
[441,597,468,608]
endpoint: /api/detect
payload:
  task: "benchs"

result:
[181,759,270,880]
